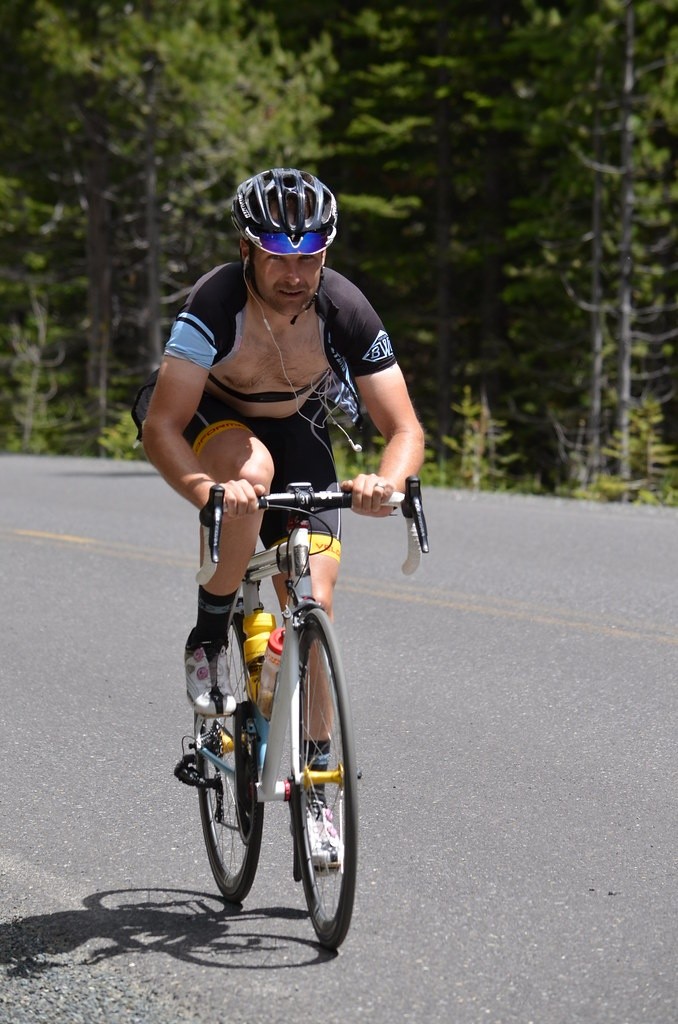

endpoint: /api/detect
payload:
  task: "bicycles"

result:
[172,477,431,951]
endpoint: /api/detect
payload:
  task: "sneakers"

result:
[185,629,236,715]
[288,801,343,867]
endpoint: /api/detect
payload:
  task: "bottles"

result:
[243,606,277,703]
[259,627,285,718]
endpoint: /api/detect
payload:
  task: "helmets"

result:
[231,167,337,256]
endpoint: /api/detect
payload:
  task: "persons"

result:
[143,168,425,870]
